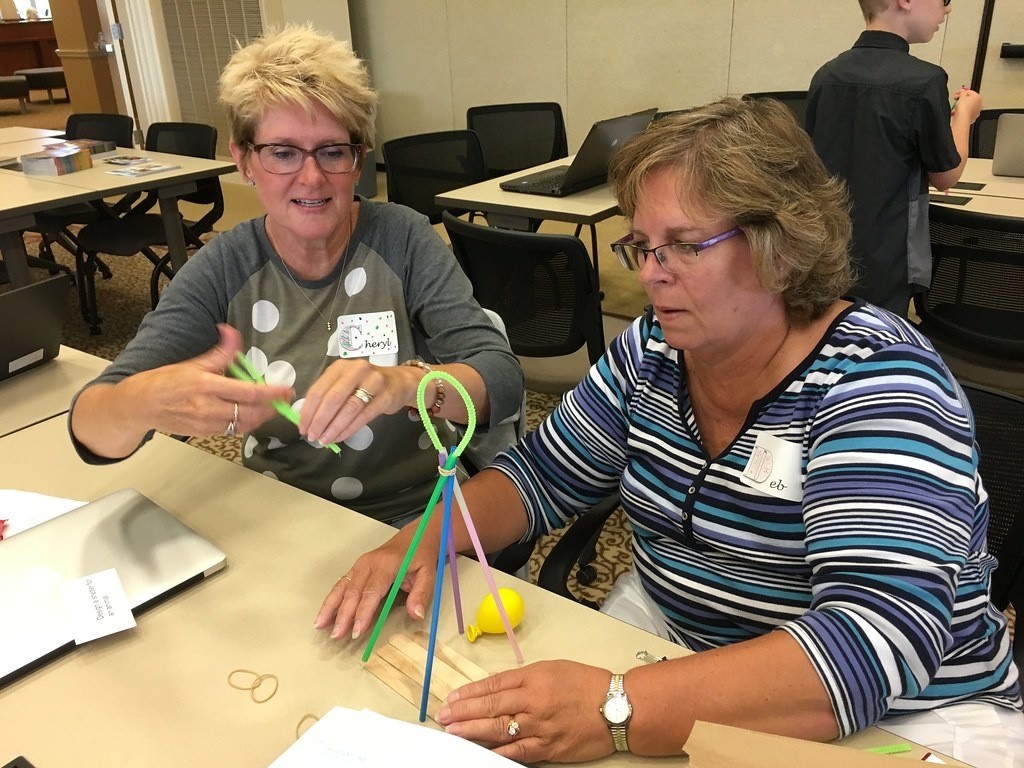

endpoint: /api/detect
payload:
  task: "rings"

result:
[343,576,351,580]
[354,388,373,403]
[234,402,238,421]
[228,421,234,431]
[507,714,520,741]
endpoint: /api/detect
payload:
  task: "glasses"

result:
[610,227,746,275]
[944,0,951,6]
[243,137,368,175]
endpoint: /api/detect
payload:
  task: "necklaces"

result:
[266,211,351,331]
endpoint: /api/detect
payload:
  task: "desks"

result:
[0,345,972,768]
[435,154,620,284]
[929,157,1024,218]
[0,126,239,290]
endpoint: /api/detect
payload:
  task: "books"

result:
[19,147,93,177]
[103,155,152,165]
[104,164,181,177]
[42,138,117,155]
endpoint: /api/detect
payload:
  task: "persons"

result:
[310,99,1024,768]
[806,0,981,318]
[69,25,524,527]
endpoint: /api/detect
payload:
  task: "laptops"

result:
[0,274,69,382]
[992,113,1024,177]
[498,107,658,197]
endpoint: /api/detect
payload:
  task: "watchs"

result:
[600,674,632,751]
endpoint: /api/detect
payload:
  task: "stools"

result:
[0,67,66,114]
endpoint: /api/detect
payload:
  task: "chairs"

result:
[0,114,225,334]
[375,89,1024,671]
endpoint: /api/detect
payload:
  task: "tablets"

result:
[1,487,227,686]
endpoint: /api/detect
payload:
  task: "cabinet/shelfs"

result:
[0,18,62,77]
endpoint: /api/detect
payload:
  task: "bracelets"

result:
[400,360,445,417]
